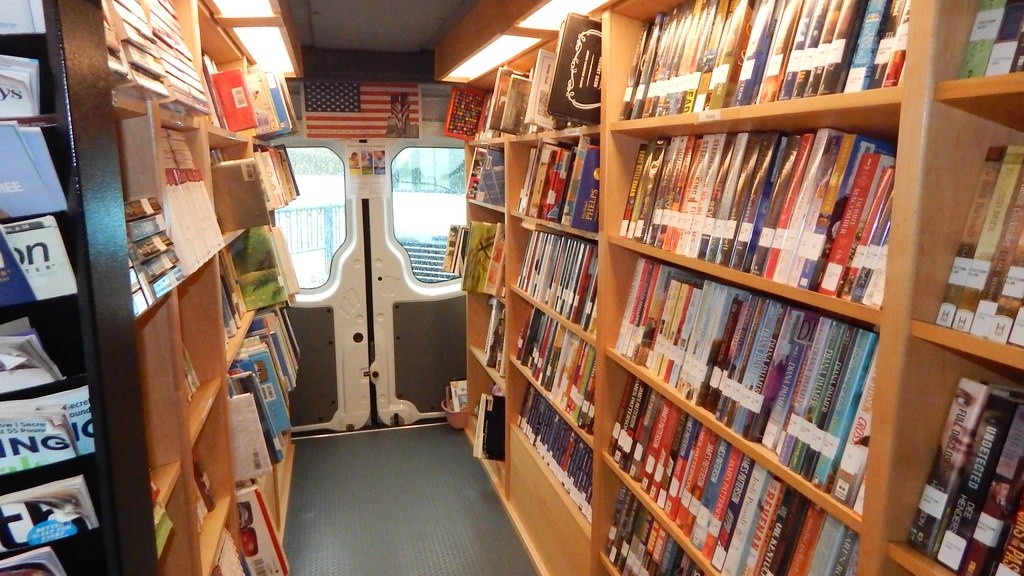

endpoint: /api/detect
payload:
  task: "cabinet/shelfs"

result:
[463,0,1024,576]
[0,0,295,576]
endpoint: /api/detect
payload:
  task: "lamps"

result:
[202,0,304,78]
[434,0,619,83]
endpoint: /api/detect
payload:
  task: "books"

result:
[0,0,1024,576]
